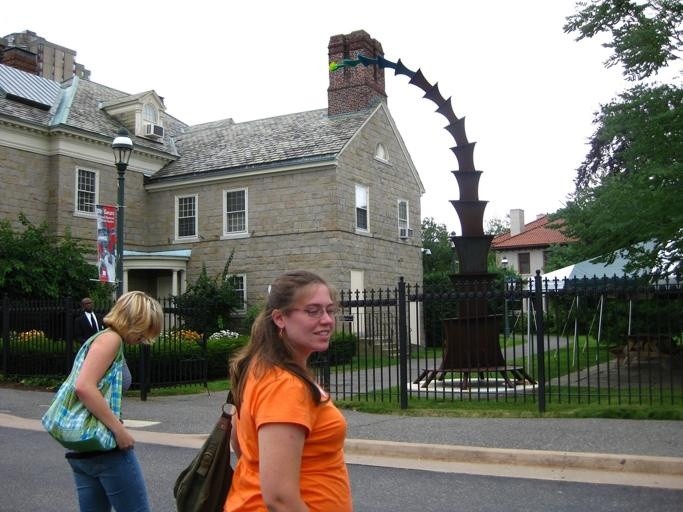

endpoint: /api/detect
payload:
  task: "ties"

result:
[90,314,98,332]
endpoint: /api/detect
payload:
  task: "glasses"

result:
[290,305,340,318]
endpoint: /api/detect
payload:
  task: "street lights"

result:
[109,125,137,307]
[500,255,511,338]
[448,230,459,278]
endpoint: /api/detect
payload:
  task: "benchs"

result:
[608,332,673,371]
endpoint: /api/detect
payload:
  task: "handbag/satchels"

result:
[173,389,236,512]
[40,330,124,452]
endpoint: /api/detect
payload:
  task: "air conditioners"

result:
[143,123,163,140]
[398,227,414,239]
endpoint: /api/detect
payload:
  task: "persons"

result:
[223,271,353,512]
[74,298,105,346]
[43,292,164,512]
